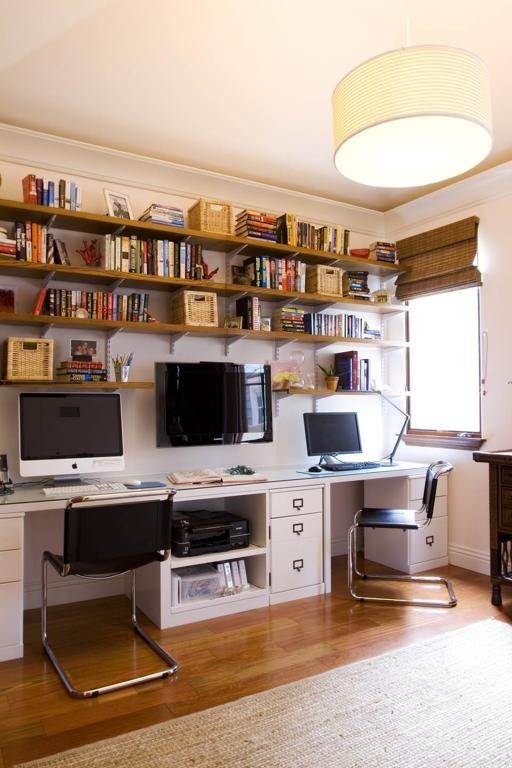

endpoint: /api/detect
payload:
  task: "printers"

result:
[172,510,249,558]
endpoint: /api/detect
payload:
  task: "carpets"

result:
[12,615,512,768]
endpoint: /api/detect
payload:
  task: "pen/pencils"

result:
[191,479,224,485]
[111,350,136,365]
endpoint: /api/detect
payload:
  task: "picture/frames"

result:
[102,188,135,220]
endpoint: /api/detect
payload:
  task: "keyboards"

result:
[43,482,128,497]
[321,462,379,471]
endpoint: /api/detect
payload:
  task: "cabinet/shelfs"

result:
[0,199,410,395]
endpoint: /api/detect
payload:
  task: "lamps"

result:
[331,0,494,189]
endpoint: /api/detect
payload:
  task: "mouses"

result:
[309,465,322,472]
[125,480,141,486]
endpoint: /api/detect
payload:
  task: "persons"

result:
[74,342,94,355]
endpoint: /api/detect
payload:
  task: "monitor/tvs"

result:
[304,412,363,465]
[156,362,272,448]
[18,391,125,488]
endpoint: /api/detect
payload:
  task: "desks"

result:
[473,449,512,605]
[0,459,449,661]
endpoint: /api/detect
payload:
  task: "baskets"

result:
[169,288,219,328]
[186,195,235,236]
[305,264,344,298]
[2,332,55,383]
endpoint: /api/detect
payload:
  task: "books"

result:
[55,360,108,384]
[334,351,360,389]
[166,466,267,486]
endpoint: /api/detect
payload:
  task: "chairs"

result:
[347,461,458,608]
[41,488,179,699]
